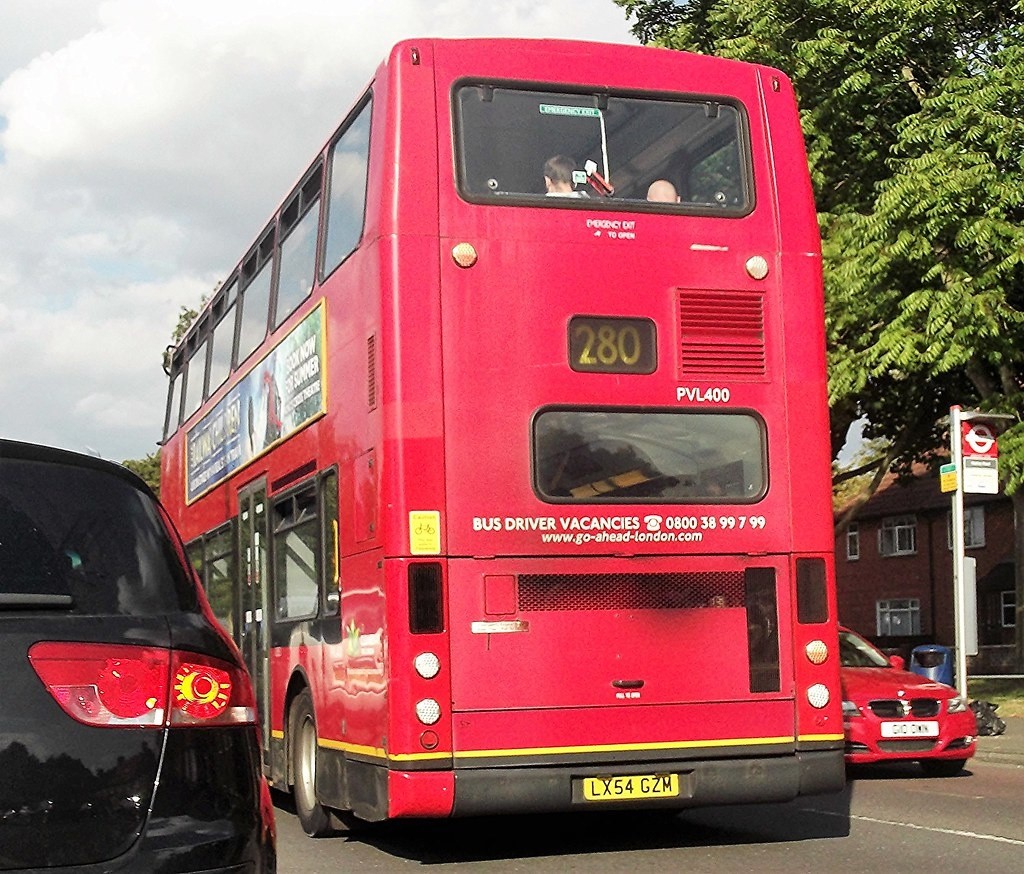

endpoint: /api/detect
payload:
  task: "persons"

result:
[646,180,681,203]
[542,154,591,199]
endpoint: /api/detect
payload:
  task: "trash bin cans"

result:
[910,644,954,686]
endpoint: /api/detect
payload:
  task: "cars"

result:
[839,624,977,776]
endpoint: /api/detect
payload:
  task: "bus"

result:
[161,38,846,839]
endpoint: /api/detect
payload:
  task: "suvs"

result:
[0,436,276,874]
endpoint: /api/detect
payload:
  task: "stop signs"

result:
[962,421,998,458]
